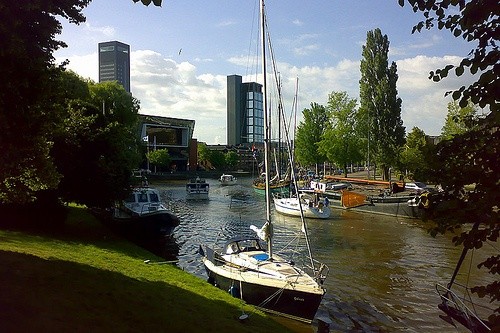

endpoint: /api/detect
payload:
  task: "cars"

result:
[405,182,434,194]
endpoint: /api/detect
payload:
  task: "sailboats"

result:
[273,77,331,219]
[200,0,329,324]
[251,72,315,190]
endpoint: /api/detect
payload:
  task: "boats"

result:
[87,169,180,241]
[433,222,500,333]
[218,174,238,185]
[186,175,209,200]
